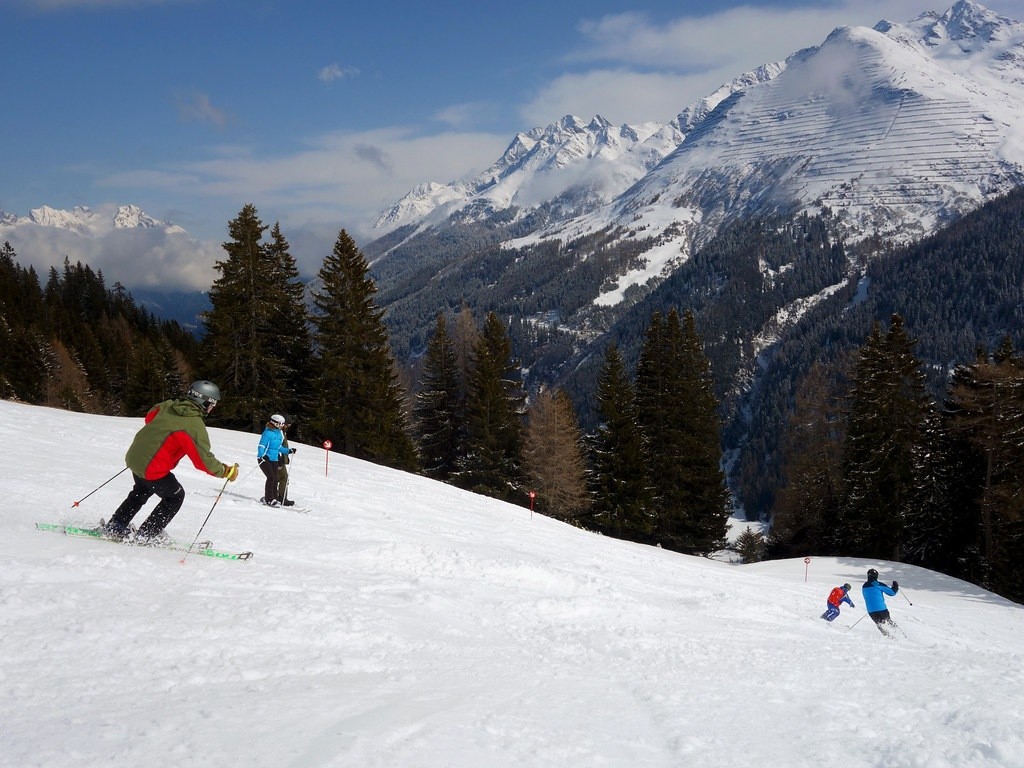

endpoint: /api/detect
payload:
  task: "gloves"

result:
[263,455,269,461]
[892,581,898,591]
[221,463,239,482]
[289,448,296,454]
[850,603,855,608]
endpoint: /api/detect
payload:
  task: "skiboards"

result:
[192,486,313,516]
[35,522,255,562]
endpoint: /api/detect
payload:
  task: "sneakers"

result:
[278,497,294,506]
[263,497,281,507]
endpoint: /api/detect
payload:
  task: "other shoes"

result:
[106,518,139,539]
[135,534,175,546]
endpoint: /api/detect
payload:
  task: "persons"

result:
[820,583,854,622]
[104,380,239,544]
[256,414,296,506]
[276,418,294,506]
[862,569,899,639]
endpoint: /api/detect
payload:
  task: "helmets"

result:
[186,380,221,409]
[270,414,286,429]
[286,416,293,424]
[868,569,878,578]
[844,584,851,590]
